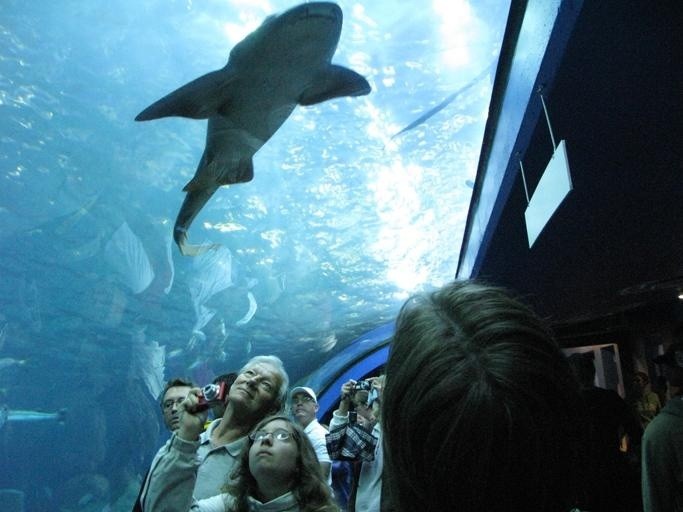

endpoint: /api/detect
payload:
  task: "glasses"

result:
[250,430,300,442]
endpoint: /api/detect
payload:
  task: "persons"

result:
[376,272,588,512]
[639,307,682,512]
[621,369,663,457]
[206,372,237,418]
[650,354,679,408]
[288,385,334,500]
[321,374,385,512]
[567,351,643,512]
[190,352,288,502]
[137,384,343,512]
[129,375,212,512]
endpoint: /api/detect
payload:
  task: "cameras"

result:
[196,382,226,410]
[354,382,371,391]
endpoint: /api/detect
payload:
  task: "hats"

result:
[657,344,683,368]
[290,386,315,402]
